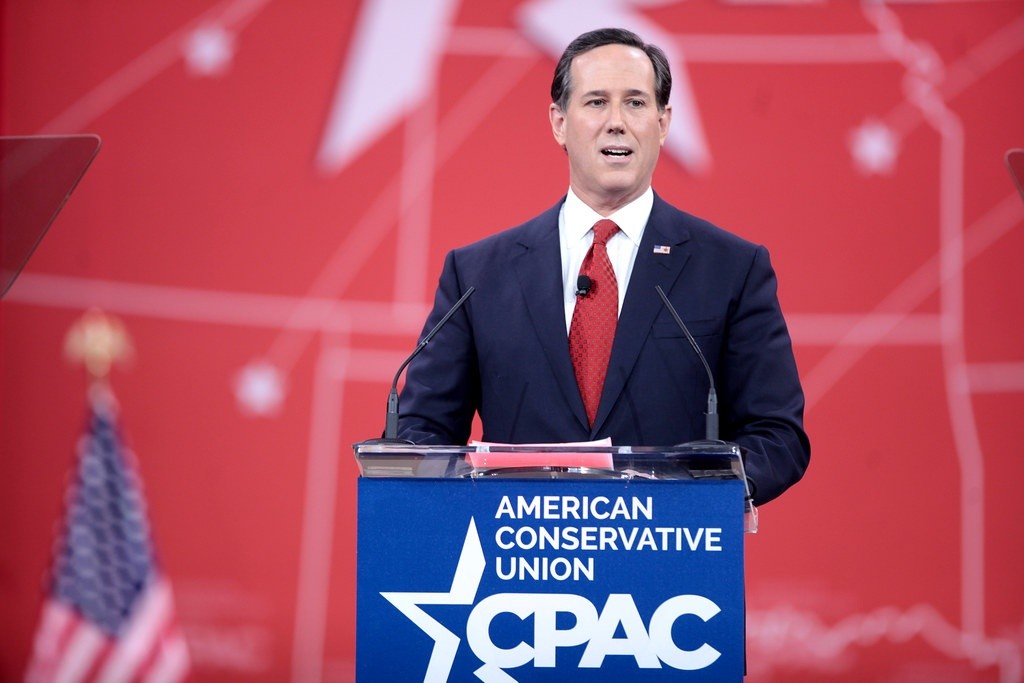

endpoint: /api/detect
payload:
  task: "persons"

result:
[380,27,812,513]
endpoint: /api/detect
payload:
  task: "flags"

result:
[20,382,196,683]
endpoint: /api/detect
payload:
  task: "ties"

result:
[569,219,620,431]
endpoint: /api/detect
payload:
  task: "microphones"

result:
[576,275,590,296]
[354,286,475,456]
[655,284,728,447]
